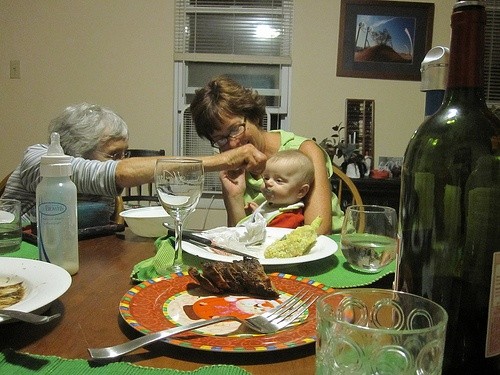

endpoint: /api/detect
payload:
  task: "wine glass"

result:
[154,157,205,276]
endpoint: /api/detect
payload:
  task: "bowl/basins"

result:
[119,205,196,238]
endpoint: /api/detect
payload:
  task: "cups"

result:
[0,198,24,256]
[315,287,449,375]
[339,204,399,274]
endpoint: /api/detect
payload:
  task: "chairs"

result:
[119,149,166,210]
[330,166,366,234]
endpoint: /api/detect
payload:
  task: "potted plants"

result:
[319,121,368,178]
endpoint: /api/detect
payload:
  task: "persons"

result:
[190,79,357,234]
[1,101,267,219]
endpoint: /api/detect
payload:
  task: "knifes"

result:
[162,221,258,260]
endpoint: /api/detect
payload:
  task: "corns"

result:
[264,216,323,259]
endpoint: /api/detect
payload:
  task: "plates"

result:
[118,268,354,353]
[180,225,339,265]
[2,241,40,260]
[0,256,73,323]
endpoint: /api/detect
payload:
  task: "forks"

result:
[87,286,320,360]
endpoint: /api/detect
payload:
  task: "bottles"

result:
[396,2,500,372]
[34,132,80,276]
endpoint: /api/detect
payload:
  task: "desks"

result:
[329,175,400,219]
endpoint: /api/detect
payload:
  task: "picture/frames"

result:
[336,0,435,81]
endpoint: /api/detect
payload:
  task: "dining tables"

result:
[0,228,401,375]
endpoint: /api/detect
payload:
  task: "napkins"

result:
[211,211,266,258]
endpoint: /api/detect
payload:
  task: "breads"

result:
[188,257,280,298]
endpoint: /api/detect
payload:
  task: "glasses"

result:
[210,114,247,150]
[94,149,131,160]
